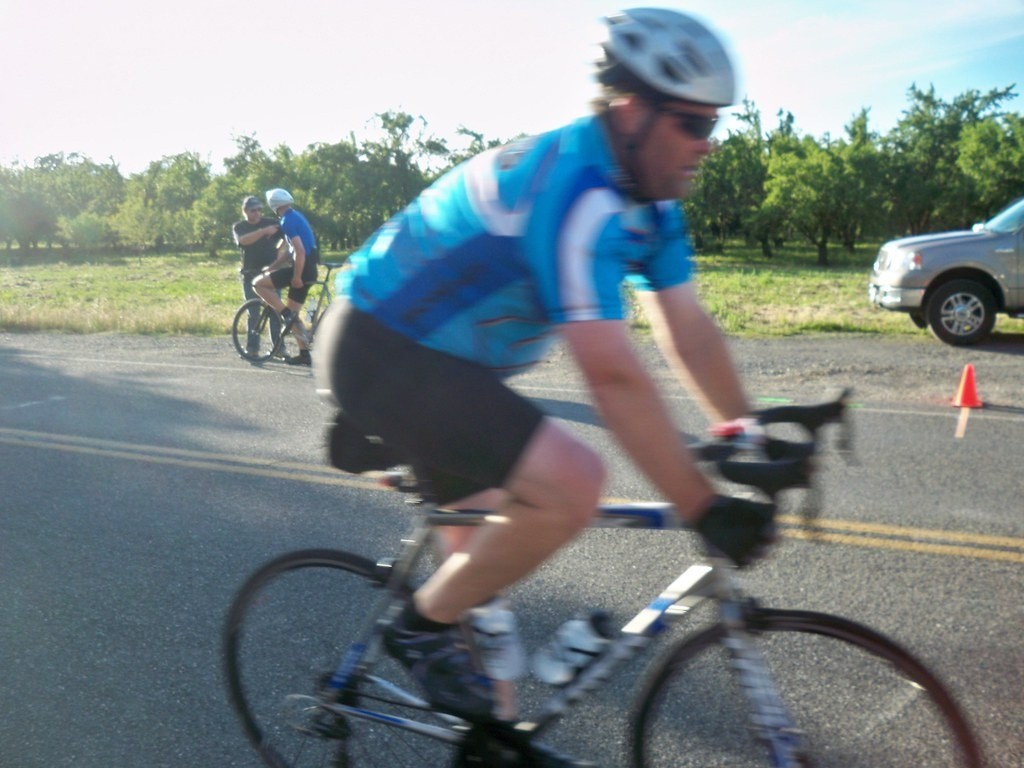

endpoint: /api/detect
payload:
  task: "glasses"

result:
[246,208,263,213]
[634,90,719,139]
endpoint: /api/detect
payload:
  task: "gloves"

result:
[758,434,815,490]
[685,493,780,570]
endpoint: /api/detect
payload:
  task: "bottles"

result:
[528,610,613,684]
[463,593,522,682]
[305,297,316,322]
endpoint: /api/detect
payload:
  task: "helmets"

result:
[592,7,734,107]
[265,188,295,210]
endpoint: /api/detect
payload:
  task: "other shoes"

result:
[447,715,596,768]
[380,595,493,725]
[284,352,311,365]
[280,308,294,324]
[274,349,290,358]
[248,350,261,359]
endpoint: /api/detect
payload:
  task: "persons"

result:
[311,7,846,728]
[233,188,318,367]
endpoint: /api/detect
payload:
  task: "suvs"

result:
[869,201,1023,347]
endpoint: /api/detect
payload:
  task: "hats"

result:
[242,196,262,206]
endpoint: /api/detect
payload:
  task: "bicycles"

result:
[232,261,342,364]
[221,381,985,768]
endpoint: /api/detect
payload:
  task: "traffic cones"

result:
[949,362,985,407]
